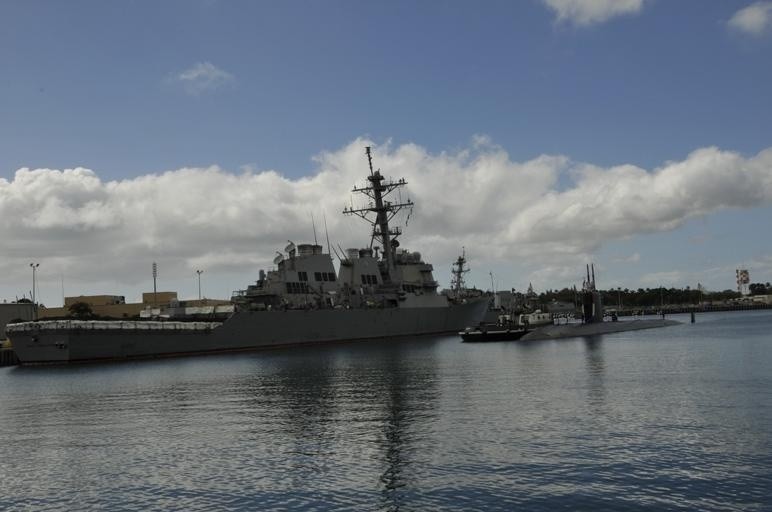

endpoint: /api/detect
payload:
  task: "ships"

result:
[7,143,492,372]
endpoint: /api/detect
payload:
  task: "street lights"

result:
[195,268,204,300]
[29,261,42,320]
[618,287,623,310]
[150,260,160,308]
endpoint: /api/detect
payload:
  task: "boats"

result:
[458,326,532,344]
